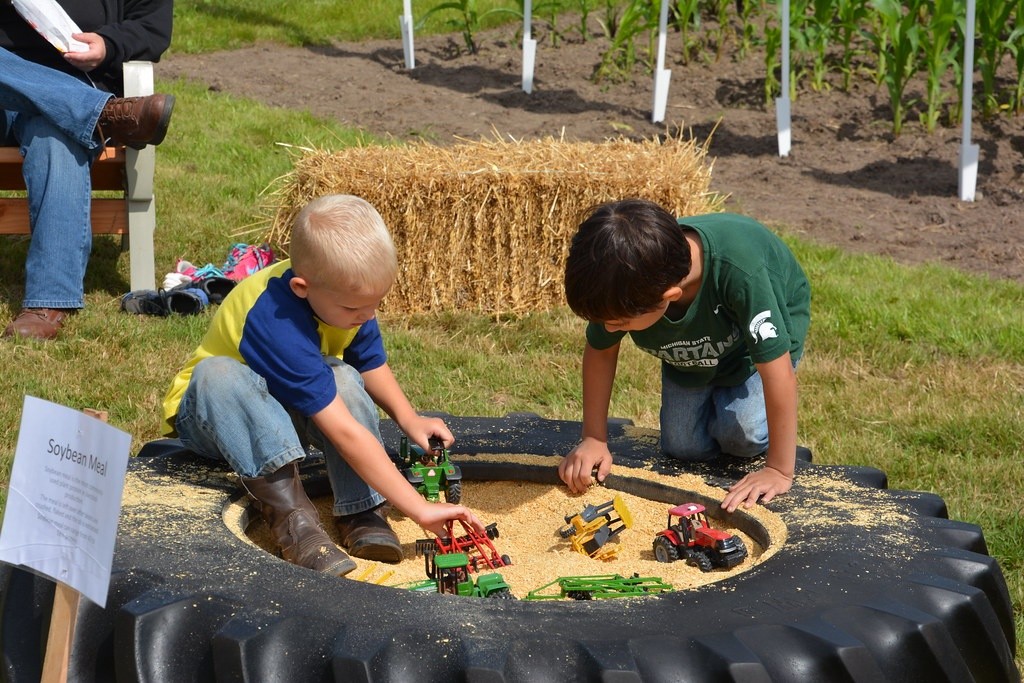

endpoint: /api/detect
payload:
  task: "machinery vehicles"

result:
[409,554,516,600]
[388,436,462,505]
[416,501,512,583]
[560,495,633,560]
[653,502,748,573]
[521,573,676,599]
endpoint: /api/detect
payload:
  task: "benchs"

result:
[1,59,156,292]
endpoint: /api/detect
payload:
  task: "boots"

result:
[331,502,405,564]
[238,462,357,577]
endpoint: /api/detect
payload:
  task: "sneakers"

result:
[176,257,225,284]
[221,241,273,282]
[120,288,209,317]
[170,278,238,308]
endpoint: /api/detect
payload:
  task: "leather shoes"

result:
[99,94,174,151]
[6,308,70,340]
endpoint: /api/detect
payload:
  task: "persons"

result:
[161,192,487,578]
[0,0,177,344]
[556,196,815,513]
